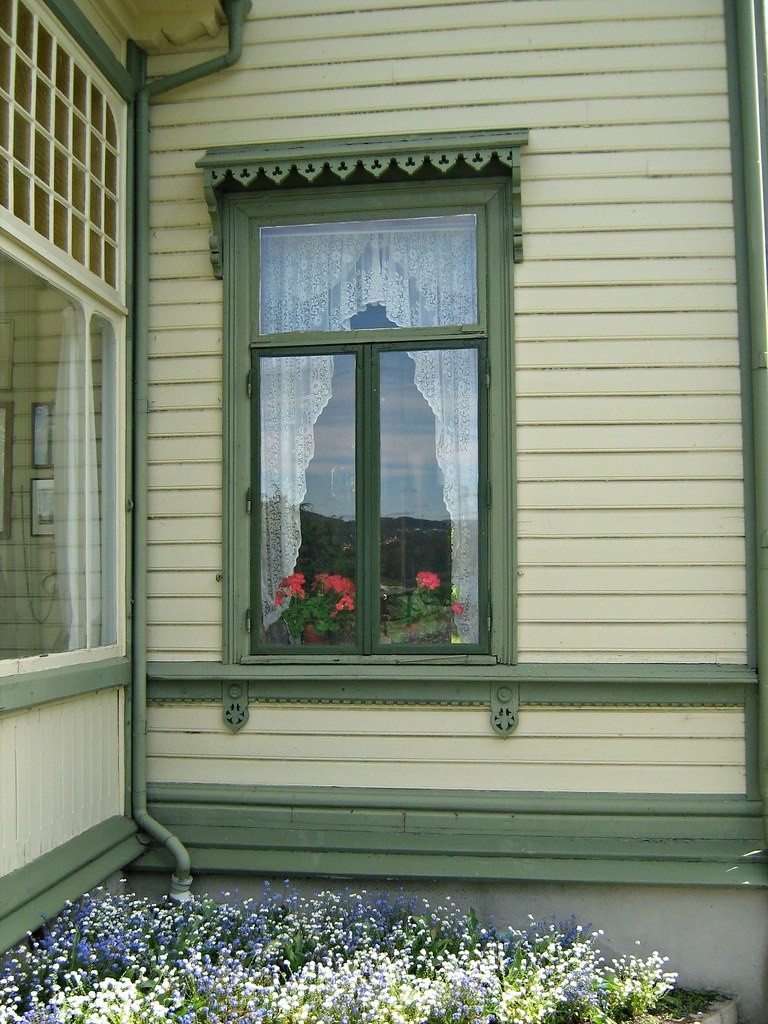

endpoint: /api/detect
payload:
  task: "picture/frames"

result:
[0,320,14,390]
[0,400,14,542]
[30,401,58,469]
[29,476,61,539]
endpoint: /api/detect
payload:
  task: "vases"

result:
[408,617,447,640]
[302,624,330,646]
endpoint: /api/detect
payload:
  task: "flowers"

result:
[390,572,463,643]
[275,573,357,639]
